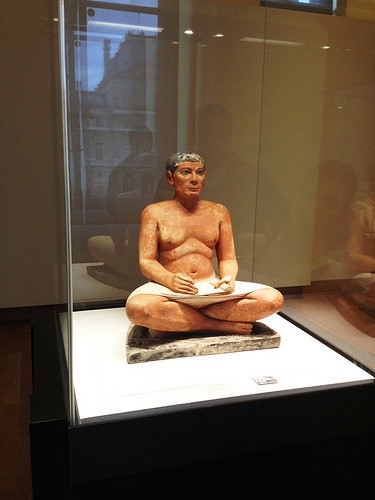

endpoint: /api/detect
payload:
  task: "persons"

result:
[126,150,283,338]
